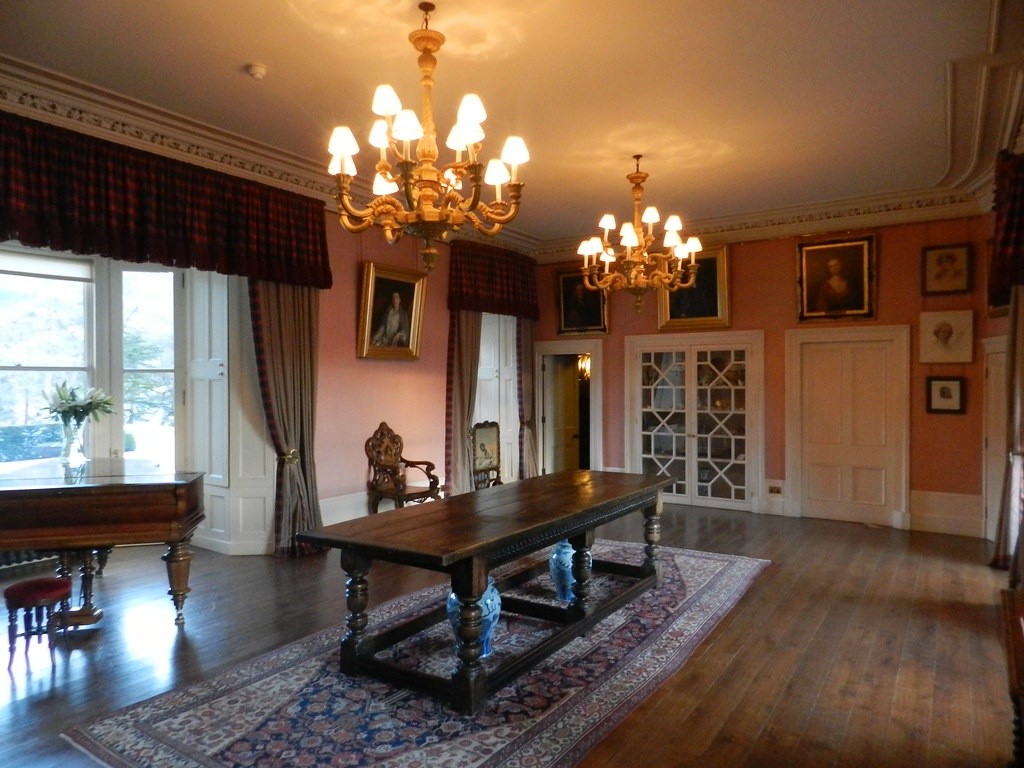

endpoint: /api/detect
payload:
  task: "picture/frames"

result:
[921,242,974,297]
[558,269,607,336]
[795,232,878,325]
[356,261,429,361]
[927,376,964,414]
[655,245,733,332]
[985,238,1010,317]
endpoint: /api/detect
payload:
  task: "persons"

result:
[705,357,739,387]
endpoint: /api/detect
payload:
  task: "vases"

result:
[447,576,501,659]
[62,420,88,470]
[550,540,592,603]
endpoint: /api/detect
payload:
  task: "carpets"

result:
[58,538,772,768]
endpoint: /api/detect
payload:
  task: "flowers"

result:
[38,381,116,426]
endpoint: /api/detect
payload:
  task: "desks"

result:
[296,467,680,717]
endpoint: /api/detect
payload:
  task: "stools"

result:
[5,576,72,670]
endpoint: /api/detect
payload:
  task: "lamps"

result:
[577,156,703,314]
[327,2,531,272]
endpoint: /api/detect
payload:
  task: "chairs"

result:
[472,421,503,490]
[365,422,441,514]
